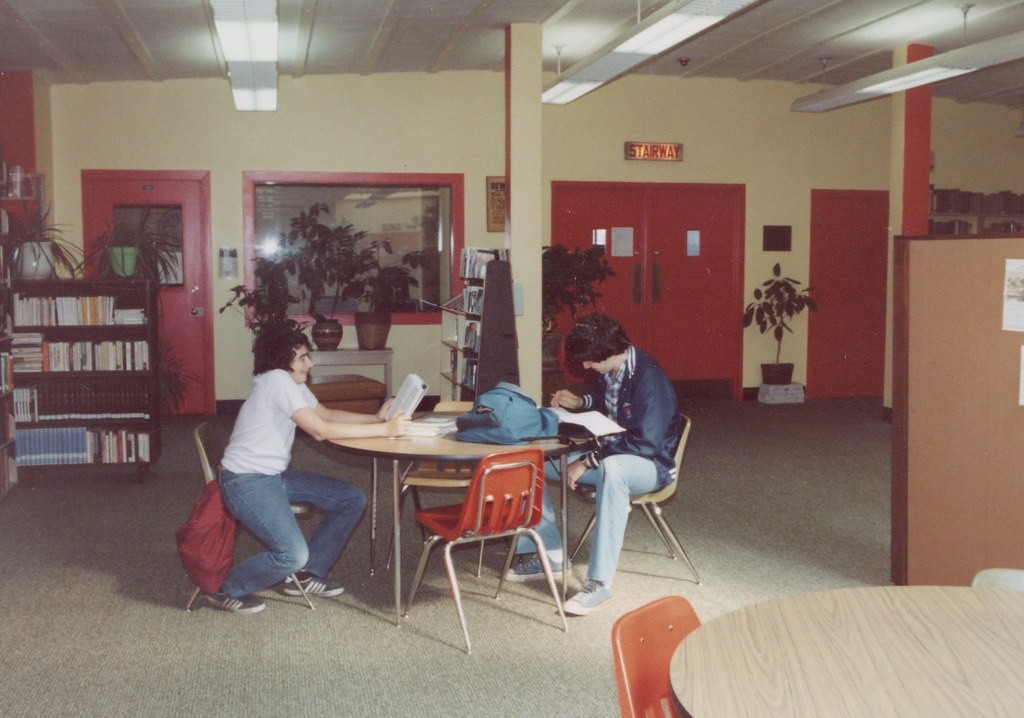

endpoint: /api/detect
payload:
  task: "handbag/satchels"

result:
[558,421,597,453]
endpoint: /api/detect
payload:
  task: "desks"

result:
[327,409,597,629]
[309,346,393,402]
[670,588,1023,718]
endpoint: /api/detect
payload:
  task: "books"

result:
[448,250,510,401]
[545,405,627,436]
[0,293,152,489]
[385,373,458,439]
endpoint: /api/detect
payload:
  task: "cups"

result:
[8,165,25,197]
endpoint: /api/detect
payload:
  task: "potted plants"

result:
[1,200,91,291]
[742,265,818,383]
[86,205,187,290]
[218,199,425,377]
[541,242,615,407]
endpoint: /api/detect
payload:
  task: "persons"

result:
[205,328,412,615]
[502,315,678,617]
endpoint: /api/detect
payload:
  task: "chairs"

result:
[401,447,567,656]
[610,593,702,718]
[385,400,475,570]
[183,417,316,614]
[570,414,702,586]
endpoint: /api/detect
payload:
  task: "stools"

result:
[308,374,386,416]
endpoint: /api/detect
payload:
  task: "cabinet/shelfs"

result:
[442,260,522,403]
[1,207,161,496]
[927,211,1024,234]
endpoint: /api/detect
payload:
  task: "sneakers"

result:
[563,581,614,615]
[205,586,266,613]
[284,576,345,597]
[504,550,574,581]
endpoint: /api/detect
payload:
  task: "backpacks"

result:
[456,382,559,445]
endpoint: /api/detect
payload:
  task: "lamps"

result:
[789,0,1024,112]
[210,1,279,113]
[538,0,762,105]
